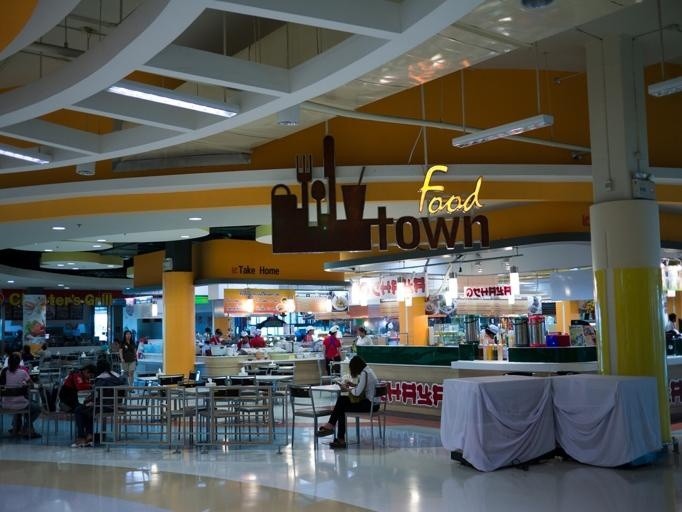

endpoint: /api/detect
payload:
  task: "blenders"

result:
[512,316,527,347]
[464,315,479,344]
[528,315,545,348]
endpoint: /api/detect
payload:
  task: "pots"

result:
[211,346,224,356]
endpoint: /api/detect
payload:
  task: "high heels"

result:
[70,442,86,449]
[86,440,94,448]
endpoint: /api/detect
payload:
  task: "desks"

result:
[285,383,358,445]
[153,356,296,445]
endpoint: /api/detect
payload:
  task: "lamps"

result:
[76,161,96,175]
[646,0,682,95]
[451,42,556,149]
[274,24,304,127]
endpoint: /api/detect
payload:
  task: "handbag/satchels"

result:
[349,370,368,404]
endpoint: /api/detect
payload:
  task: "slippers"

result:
[329,438,347,448]
[316,426,333,436]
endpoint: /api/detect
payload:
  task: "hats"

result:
[307,326,315,331]
[330,328,339,332]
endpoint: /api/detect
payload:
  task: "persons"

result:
[10,330,24,351]
[119,330,137,393]
[227,328,232,339]
[211,328,223,345]
[203,328,212,344]
[355,326,374,346]
[107,337,121,376]
[236,331,251,351]
[315,355,382,449]
[250,329,265,348]
[21,346,34,362]
[334,325,344,345]
[323,327,342,386]
[484,324,500,345]
[665,312,679,332]
[1,352,43,440]
[3,347,25,368]
[37,342,51,363]
[303,325,315,342]
[384,317,400,332]
[58,363,98,439]
[71,353,129,449]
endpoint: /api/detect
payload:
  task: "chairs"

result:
[92,352,293,451]
[345,384,388,450]
[286,384,336,452]
[37,386,77,444]
[1,384,31,441]
[317,359,338,400]
